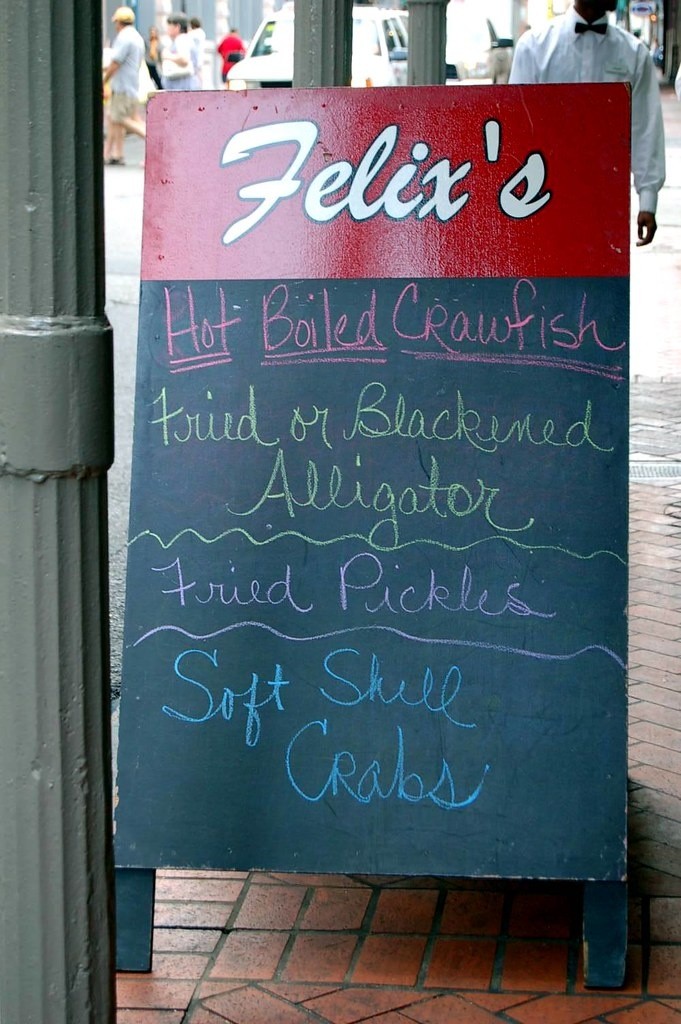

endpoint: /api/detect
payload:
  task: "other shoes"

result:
[104,156,125,165]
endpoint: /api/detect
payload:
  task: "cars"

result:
[447,16,514,85]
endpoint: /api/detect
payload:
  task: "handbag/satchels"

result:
[227,53,243,62]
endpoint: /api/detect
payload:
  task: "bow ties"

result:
[574,22,607,35]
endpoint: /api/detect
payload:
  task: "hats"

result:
[111,7,135,23]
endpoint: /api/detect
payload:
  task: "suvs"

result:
[226,8,409,89]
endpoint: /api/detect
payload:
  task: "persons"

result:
[144,13,205,90]
[102,8,155,166]
[217,28,247,91]
[509,0,665,246]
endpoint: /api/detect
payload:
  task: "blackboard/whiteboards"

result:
[114,278,631,885]
